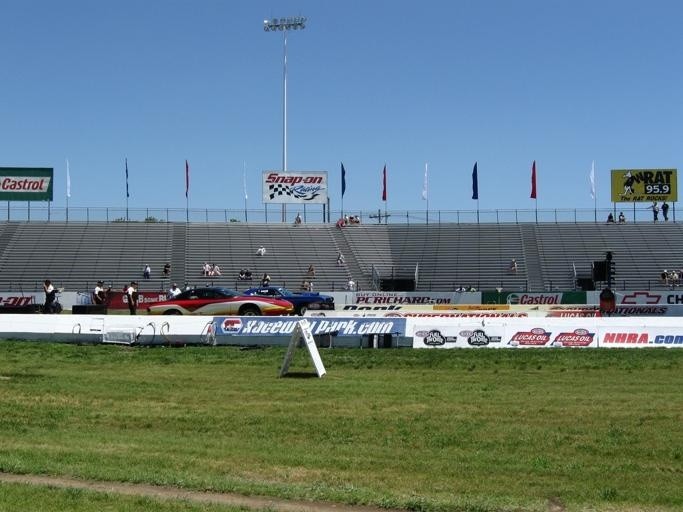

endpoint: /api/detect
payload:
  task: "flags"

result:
[421,163,427,200]
[185,159,188,196]
[529,160,535,198]
[66,156,71,198]
[586,161,594,200]
[382,164,386,201]
[471,161,478,200]
[242,162,247,198]
[339,162,347,198]
[126,159,131,196]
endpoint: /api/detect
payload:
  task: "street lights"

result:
[260,14,308,222]
[602,250,616,290]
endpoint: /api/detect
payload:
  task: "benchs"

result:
[340,218,682,294]
[0,215,358,297]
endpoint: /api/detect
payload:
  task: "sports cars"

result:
[148,286,294,316]
[241,284,336,315]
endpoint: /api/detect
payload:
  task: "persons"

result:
[255,245,265,255]
[508,259,516,274]
[43,280,60,314]
[343,277,354,290]
[125,282,138,315]
[336,251,344,263]
[162,263,170,278]
[199,262,315,292]
[335,214,361,227]
[454,285,475,292]
[646,202,660,222]
[606,213,616,224]
[662,267,682,287]
[94,279,106,310]
[294,211,303,225]
[618,212,625,223]
[660,200,669,221]
[106,281,191,299]
[143,263,150,279]
